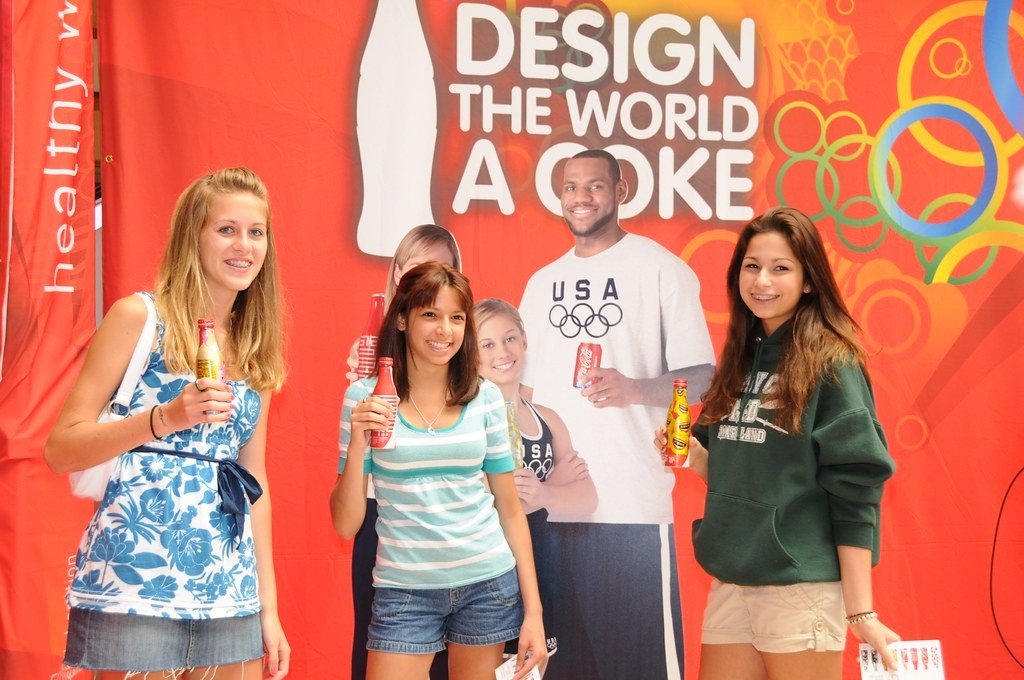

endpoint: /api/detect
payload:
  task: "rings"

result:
[194,379,204,391]
[363,397,367,402]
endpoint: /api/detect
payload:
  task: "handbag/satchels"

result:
[68,290,158,502]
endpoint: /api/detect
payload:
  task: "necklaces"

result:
[409,391,451,435]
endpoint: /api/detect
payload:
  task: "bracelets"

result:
[846,612,878,625]
[150,405,162,440]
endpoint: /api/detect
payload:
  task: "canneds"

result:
[573,342,603,390]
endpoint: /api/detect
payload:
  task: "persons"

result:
[44,166,291,680]
[517,150,716,680]
[654,207,901,680]
[472,299,599,680]
[345,223,461,680]
[329,262,548,680]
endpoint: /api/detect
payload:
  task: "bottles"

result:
[357,294,386,380]
[195,317,226,423]
[664,379,690,468]
[369,357,397,451]
